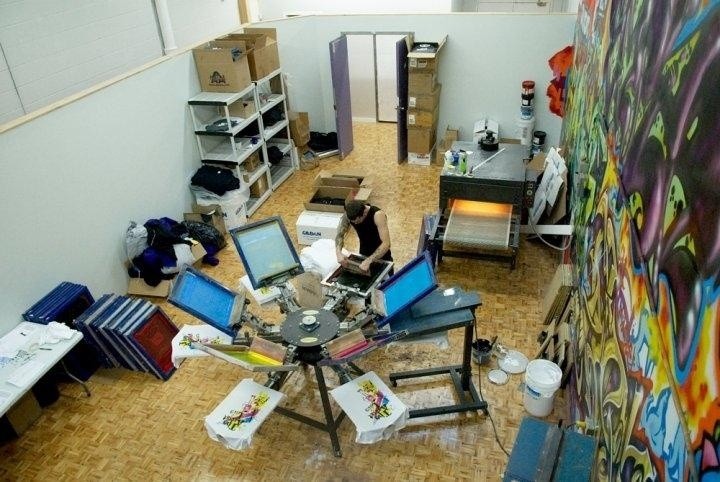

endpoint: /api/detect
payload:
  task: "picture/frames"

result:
[229,215,305,289]
[374,250,439,328]
[22,282,187,381]
[321,252,394,299]
[316,330,407,366]
[191,341,299,372]
[167,263,246,337]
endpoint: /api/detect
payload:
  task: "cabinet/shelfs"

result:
[188,69,297,217]
[427,208,522,270]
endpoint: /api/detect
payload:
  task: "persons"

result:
[335,199,394,284]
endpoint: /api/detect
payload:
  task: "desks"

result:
[440,141,537,225]
[0,321,90,418]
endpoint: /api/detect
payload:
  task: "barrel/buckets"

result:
[523,359,563,417]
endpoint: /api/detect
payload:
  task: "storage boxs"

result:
[125,237,208,297]
[183,202,227,236]
[289,112,310,146]
[405,32,460,166]
[215,28,280,80]
[473,118,499,141]
[290,271,329,309]
[250,172,268,198]
[296,168,373,245]
[191,41,255,92]
